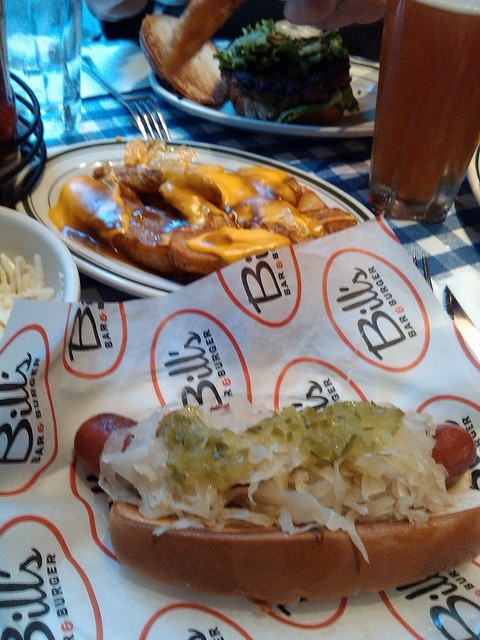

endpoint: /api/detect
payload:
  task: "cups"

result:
[370,0,480,224]
[0,0,85,147]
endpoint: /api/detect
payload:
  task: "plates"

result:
[14,138,380,305]
[467,143,480,206]
[148,37,382,138]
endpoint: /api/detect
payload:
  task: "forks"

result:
[413,253,435,293]
[79,55,171,143]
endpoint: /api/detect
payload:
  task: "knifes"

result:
[443,285,480,360]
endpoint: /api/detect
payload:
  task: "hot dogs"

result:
[76,401,480,595]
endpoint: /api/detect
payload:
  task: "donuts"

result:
[49,139,361,277]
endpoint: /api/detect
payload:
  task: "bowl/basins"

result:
[0,205,81,341]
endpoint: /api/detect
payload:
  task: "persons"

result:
[281,1,392,31]
